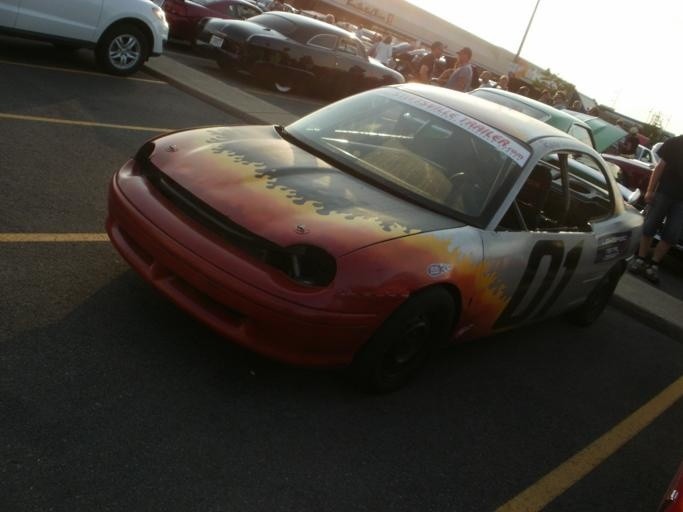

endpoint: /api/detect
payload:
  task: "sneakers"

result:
[641,259,659,283]
[627,256,646,274]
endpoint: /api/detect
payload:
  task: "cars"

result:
[196,11,404,94]
[601,144,658,192]
[106,81,645,395]
[394,87,629,178]
[1,0,169,76]
[153,0,264,48]
[281,3,447,77]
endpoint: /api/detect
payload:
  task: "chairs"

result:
[490,157,554,233]
